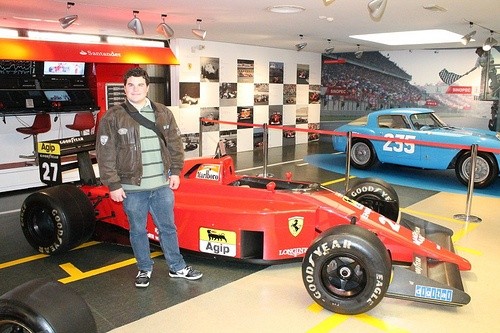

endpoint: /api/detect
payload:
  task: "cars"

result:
[331,108,499,189]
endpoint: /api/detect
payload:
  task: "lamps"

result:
[324,39,335,53]
[58,2,79,29]
[354,44,364,58]
[482,29,497,51]
[296,34,307,51]
[192,18,207,39]
[368,0,387,21]
[157,13,174,37]
[460,21,477,45]
[127,10,144,35]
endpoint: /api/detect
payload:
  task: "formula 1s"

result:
[181,63,319,150]
[15,110,471,315]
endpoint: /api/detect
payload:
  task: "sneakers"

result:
[168,266,203,280]
[135,266,153,287]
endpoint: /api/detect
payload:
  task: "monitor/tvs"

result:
[44,90,72,101]
[43,61,85,75]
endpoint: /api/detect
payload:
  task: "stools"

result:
[65,111,95,136]
[16,113,52,165]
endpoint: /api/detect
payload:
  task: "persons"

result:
[95,66,203,287]
[319,50,489,116]
[474,47,497,98]
